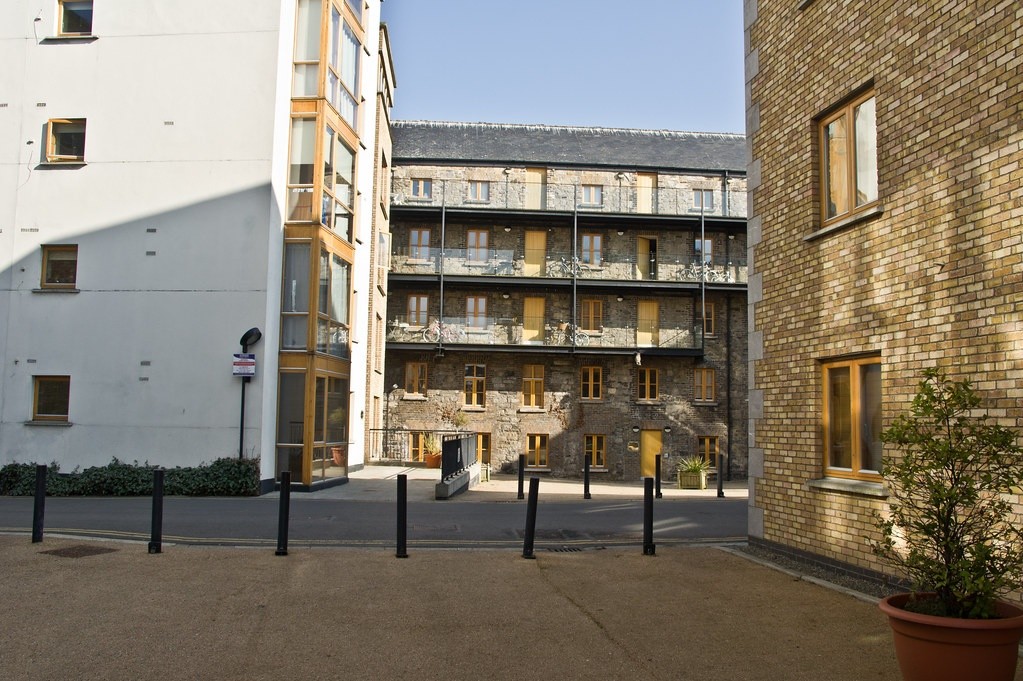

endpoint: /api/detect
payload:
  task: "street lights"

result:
[386,384,398,457]
[240,328,262,459]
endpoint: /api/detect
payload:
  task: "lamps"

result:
[617,229,625,235]
[504,224,512,232]
[728,234,735,240]
[665,426,671,432]
[632,426,639,432]
[502,291,510,299]
[617,171,625,178]
[617,295,624,302]
[504,167,511,174]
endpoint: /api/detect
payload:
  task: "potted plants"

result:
[676,454,714,489]
[418,431,442,470]
[867,366,1023,681]
